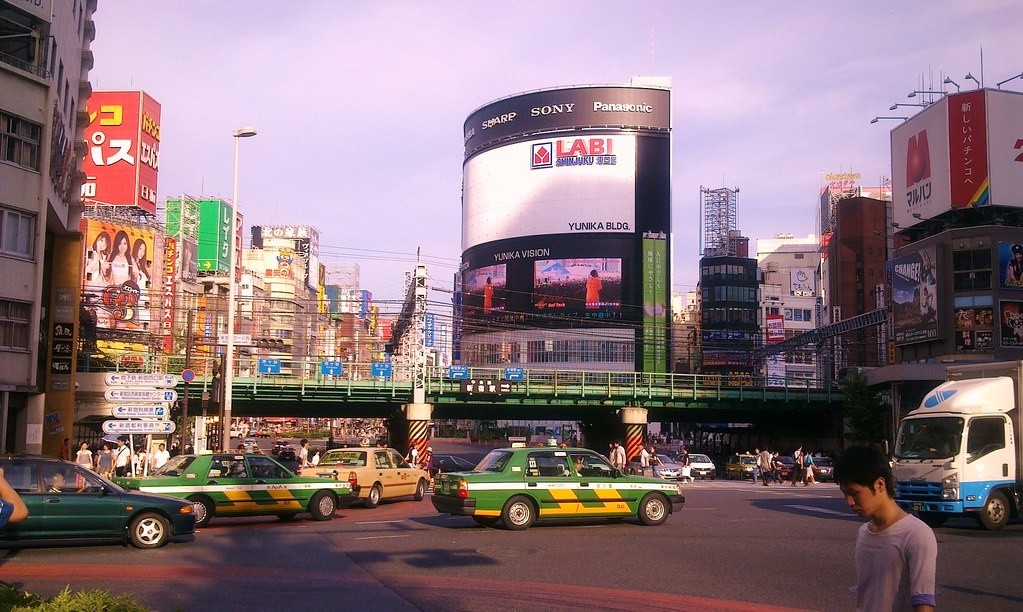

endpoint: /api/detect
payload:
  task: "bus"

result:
[839,365,879,391]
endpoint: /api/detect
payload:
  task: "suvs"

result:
[0,451,196,549]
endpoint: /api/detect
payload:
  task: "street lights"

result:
[222,127,260,457]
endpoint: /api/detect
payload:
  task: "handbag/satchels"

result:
[297,456,303,465]
[652,460,658,465]
[794,462,801,468]
[803,456,811,467]
[681,467,690,476]
[136,463,143,474]
[643,467,653,477]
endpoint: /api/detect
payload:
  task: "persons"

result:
[920,287,938,324]
[0,467,29,528]
[84,230,151,331]
[47,436,436,495]
[833,441,939,611]
[1006,244,1023,286]
[585,270,603,308]
[485,277,494,316]
[539,440,823,488]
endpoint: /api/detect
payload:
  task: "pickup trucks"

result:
[726,454,759,480]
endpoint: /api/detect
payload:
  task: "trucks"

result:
[881,361,1023,533]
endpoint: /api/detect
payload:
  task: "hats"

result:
[411,441,418,447]
[1011,244,1023,252]
[426,447,432,452]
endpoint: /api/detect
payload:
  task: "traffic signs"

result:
[449,366,468,379]
[103,372,179,435]
[372,364,391,376]
[505,366,523,380]
[322,361,342,375]
[258,360,281,374]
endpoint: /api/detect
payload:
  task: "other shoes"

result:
[750,479,821,489]
[691,477,694,483]
[682,481,687,483]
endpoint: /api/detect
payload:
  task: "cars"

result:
[813,457,834,482]
[589,455,614,471]
[208,423,387,460]
[678,453,717,480]
[111,451,352,527]
[431,436,686,532]
[298,446,430,508]
[780,456,794,478]
[629,454,682,480]
